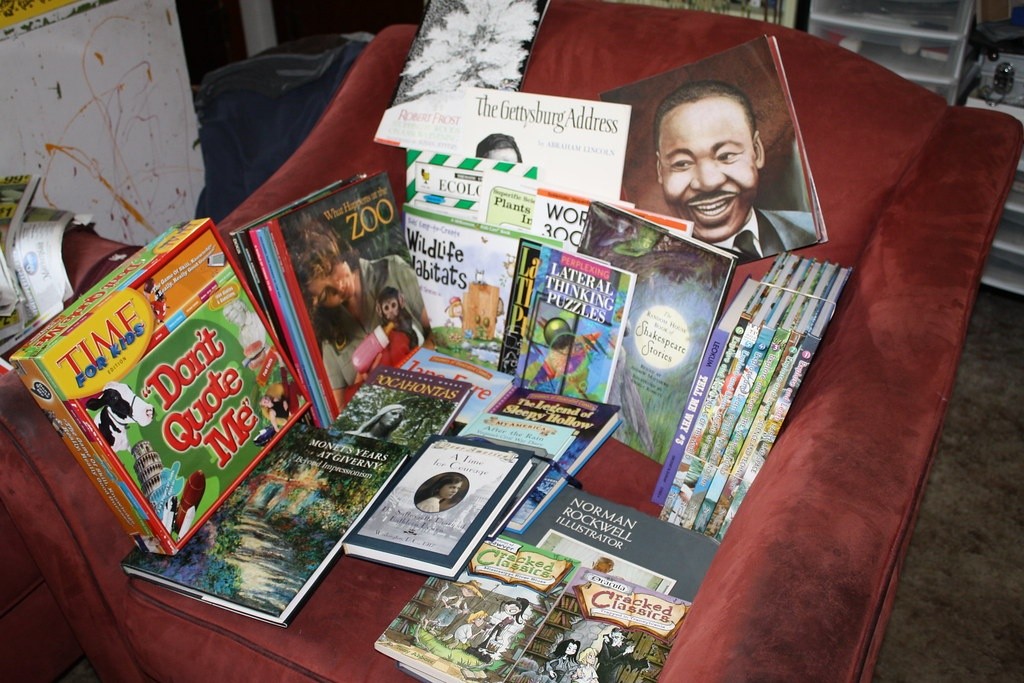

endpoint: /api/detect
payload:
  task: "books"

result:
[712,263,851,545]
[453,385,623,535]
[529,184,695,254]
[393,344,516,437]
[651,272,760,506]
[403,146,544,235]
[503,564,694,683]
[392,484,722,683]
[666,252,803,527]
[119,421,414,629]
[227,168,440,430]
[575,200,739,466]
[657,249,790,523]
[497,236,611,377]
[702,259,839,539]
[401,201,578,370]
[690,255,825,535]
[373,533,581,683]
[455,410,577,542]
[328,362,474,461]
[596,34,829,267]
[454,86,632,200]
[341,433,539,583]
[677,255,814,531]
[373,0,552,158]
[512,242,639,405]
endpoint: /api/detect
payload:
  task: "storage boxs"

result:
[8,217,313,558]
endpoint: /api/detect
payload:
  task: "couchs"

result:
[1,1,1024,683]
